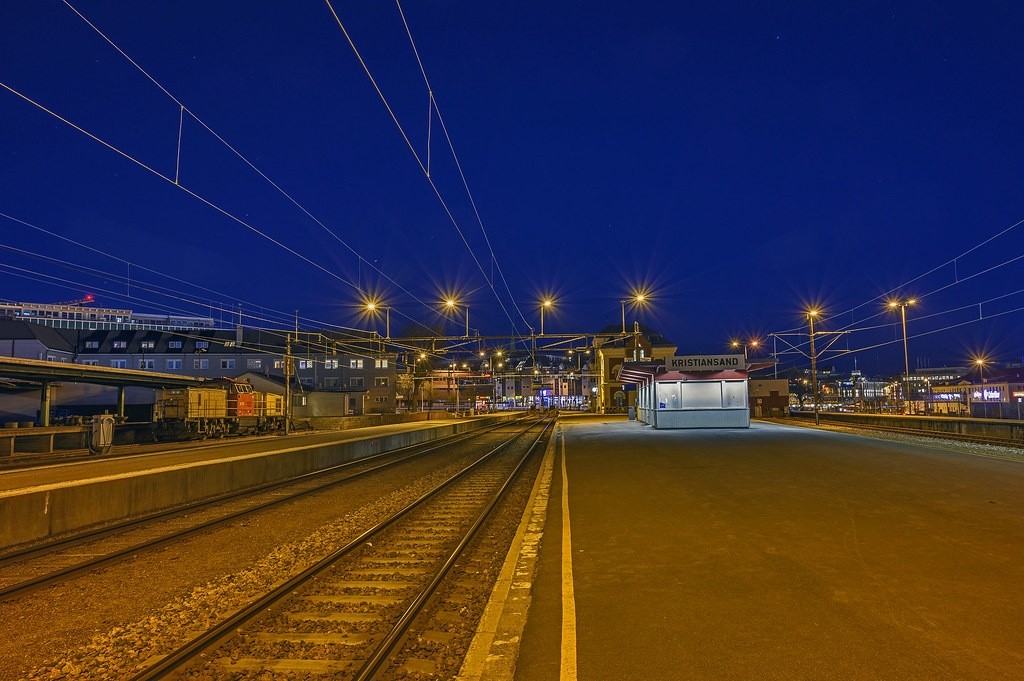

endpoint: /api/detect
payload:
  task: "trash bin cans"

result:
[628,406,635,420]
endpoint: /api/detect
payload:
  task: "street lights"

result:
[620,295,644,335]
[887,298,916,416]
[539,300,551,336]
[974,357,986,418]
[410,345,592,378]
[445,299,469,338]
[806,309,820,426]
[731,340,761,360]
[365,303,391,340]
